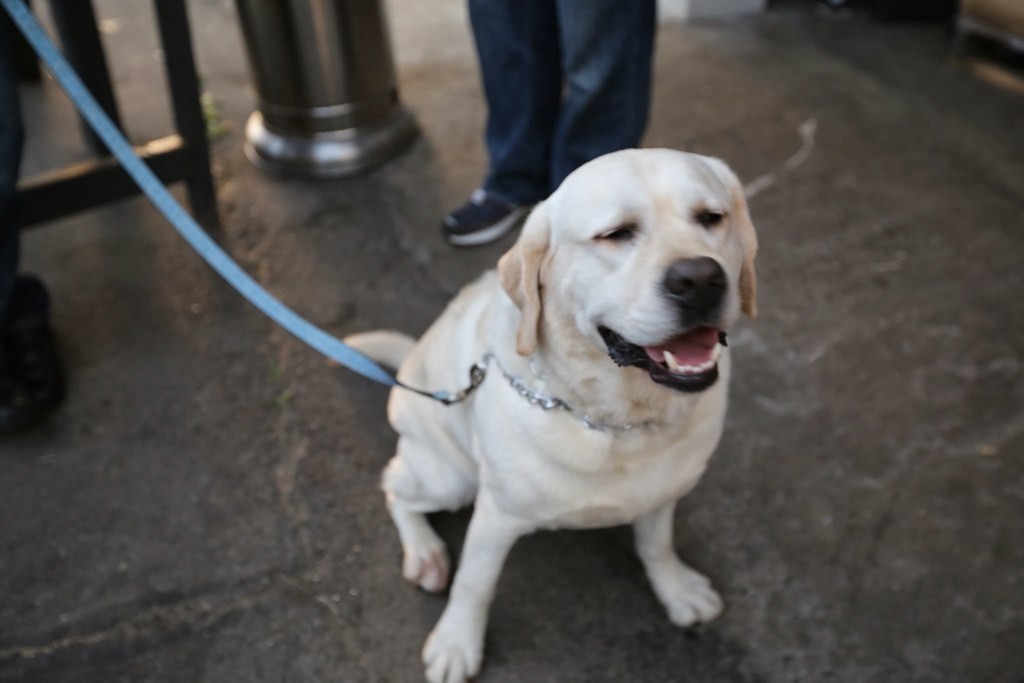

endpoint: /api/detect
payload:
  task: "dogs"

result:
[326,147,760,683]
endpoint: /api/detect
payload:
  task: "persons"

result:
[434,1,661,250]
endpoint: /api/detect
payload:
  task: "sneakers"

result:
[441,188,528,246]
